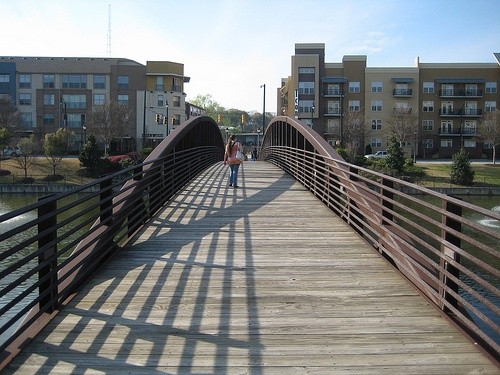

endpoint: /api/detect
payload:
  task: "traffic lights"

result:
[163,116,167,125]
[171,117,176,125]
[156,114,160,122]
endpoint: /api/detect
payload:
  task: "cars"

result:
[364,151,392,159]
[0,144,24,157]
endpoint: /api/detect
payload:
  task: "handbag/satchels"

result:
[236,150,244,163]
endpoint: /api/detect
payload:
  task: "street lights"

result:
[311,102,315,129]
[260,83,264,137]
[257,127,260,153]
[165,102,170,138]
[83,125,87,145]
[59,100,66,128]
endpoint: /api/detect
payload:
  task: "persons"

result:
[223,135,243,187]
[253,147,257,161]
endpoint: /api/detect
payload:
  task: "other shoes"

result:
[229,183,232,186]
[234,185,239,188]
